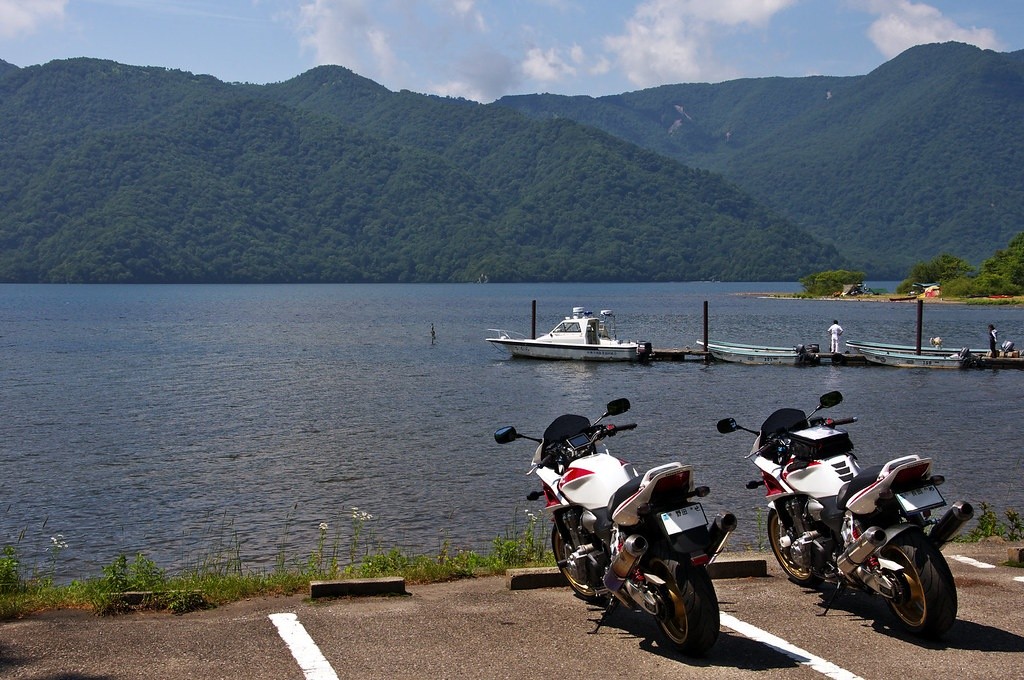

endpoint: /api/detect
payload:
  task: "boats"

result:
[978,350,1024,366]
[846,337,975,371]
[692,334,822,367]
[490,309,651,362]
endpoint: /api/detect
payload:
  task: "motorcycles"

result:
[716,389,975,645]
[496,409,739,652]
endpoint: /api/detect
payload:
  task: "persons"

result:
[828,320,844,353]
[988,324,998,358]
